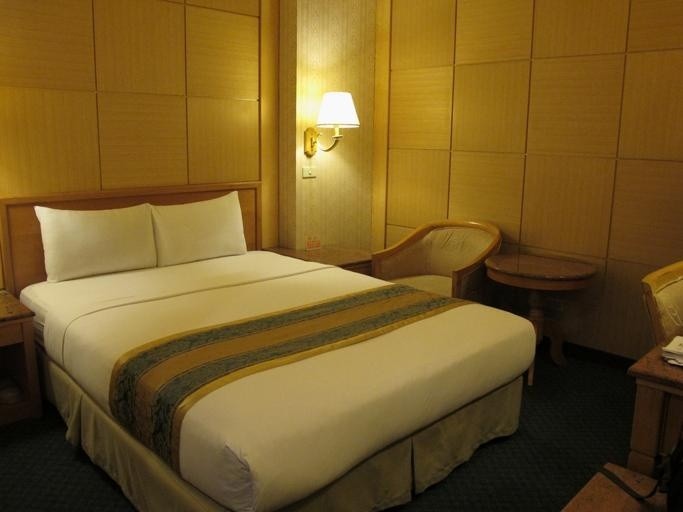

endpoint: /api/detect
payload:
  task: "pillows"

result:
[32,189,249,283]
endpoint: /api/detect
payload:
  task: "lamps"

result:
[304,92,363,158]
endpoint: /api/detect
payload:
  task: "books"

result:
[661,335,682,366]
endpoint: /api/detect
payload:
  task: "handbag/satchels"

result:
[600,433,683,512]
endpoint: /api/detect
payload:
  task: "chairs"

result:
[369,219,503,300]
[640,260,683,346]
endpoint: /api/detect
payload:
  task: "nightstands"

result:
[0,289,44,425]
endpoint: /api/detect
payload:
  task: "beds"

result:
[0,181,538,512]
